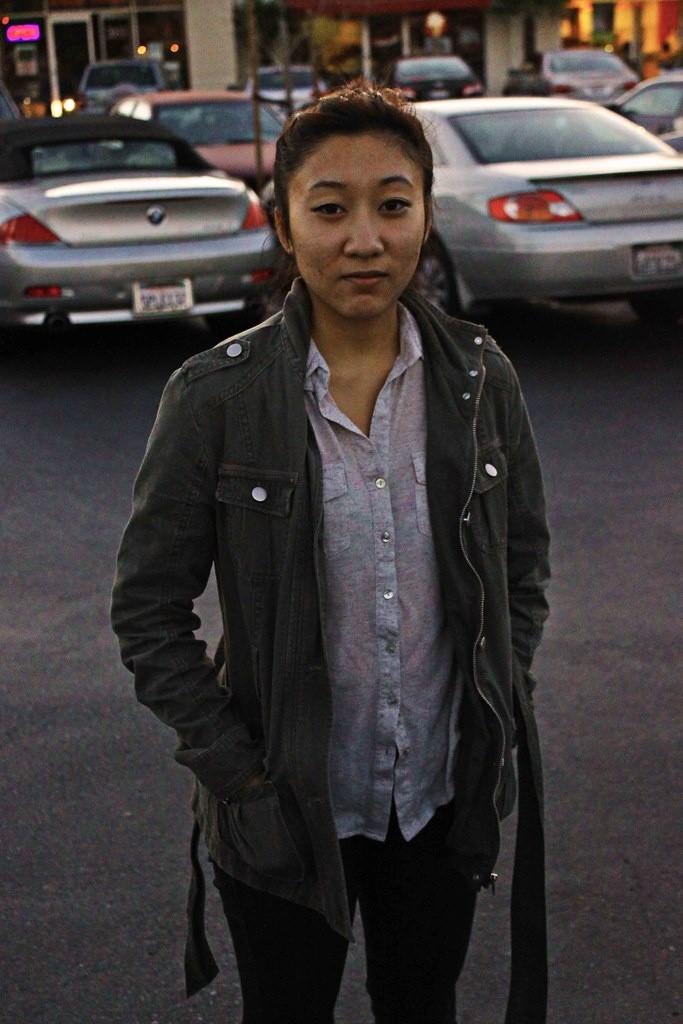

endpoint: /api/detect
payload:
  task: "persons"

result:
[657,42,673,70]
[111,85,552,1024]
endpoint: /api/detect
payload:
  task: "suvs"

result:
[72,57,175,117]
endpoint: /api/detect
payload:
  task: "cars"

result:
[0,76,46,172]
[95,86,293,193]
[376,55,487,104]
[603,69,682,153]
[533,47,644,105]
[225,61,337,115]
[252,95,682,329]
[0,110,286,342]
